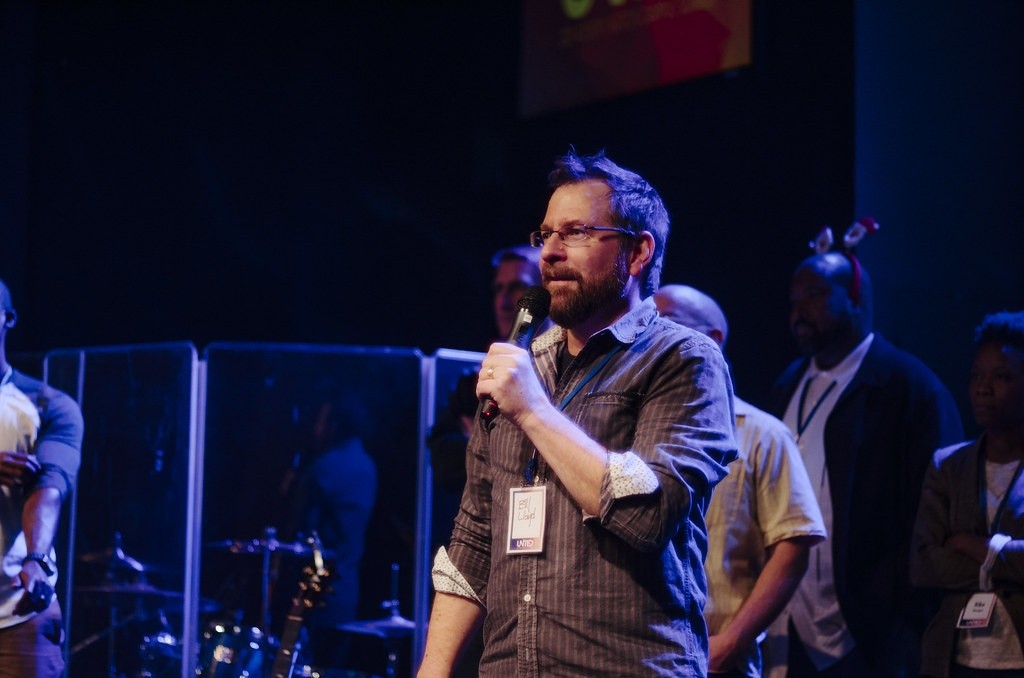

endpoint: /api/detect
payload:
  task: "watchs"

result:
[20,552,56,577]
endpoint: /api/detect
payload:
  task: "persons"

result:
[421,242,554,549]
[655,285,827,678]
[0,282,85,678]
[758,248,984,678]
[911,303,1023,678]
[414,149,742,677]
[284,401,374,666]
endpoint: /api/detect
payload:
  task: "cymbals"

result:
[333,611,418,638]
[69,581,157,596]
[202,536,311,554]
[79,549,144,571]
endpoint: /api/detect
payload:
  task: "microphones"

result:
[479,285,552,423]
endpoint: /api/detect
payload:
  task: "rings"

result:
[488,366,494,379]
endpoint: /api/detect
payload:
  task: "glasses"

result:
[530,223,636,246]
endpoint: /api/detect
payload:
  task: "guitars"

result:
[271,556,338,678]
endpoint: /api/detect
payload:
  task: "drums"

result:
[195,620,302,678]
[132,634,181,678]
